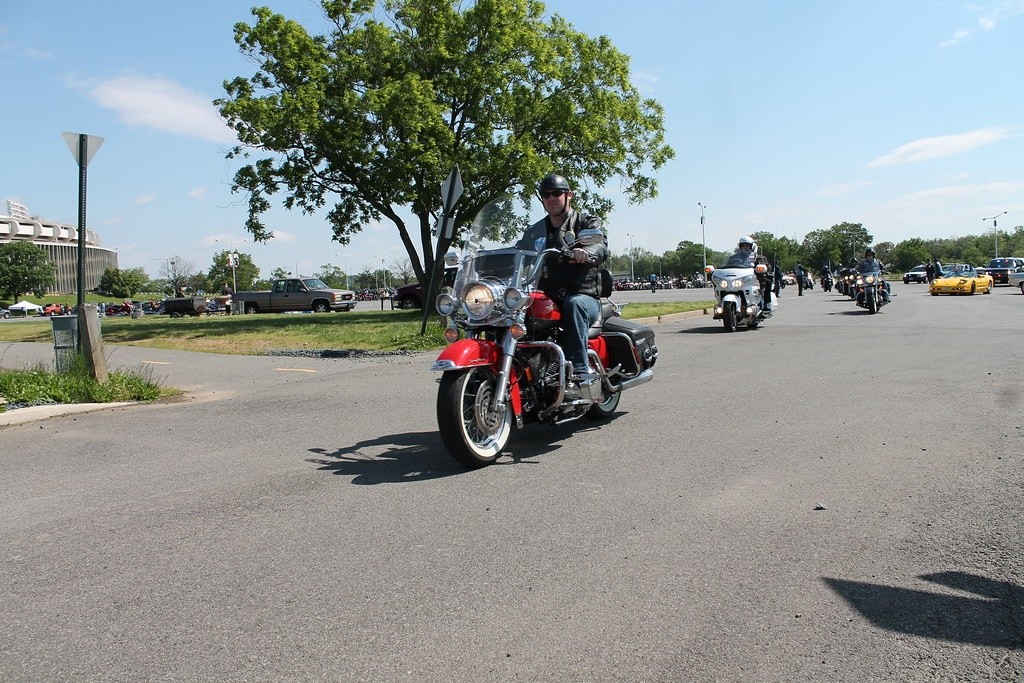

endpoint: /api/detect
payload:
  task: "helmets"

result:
[851,258,858,264]
[865,248,876,259]
[541,175,571,191]
[823,266,829,271]
[738,236,758,255]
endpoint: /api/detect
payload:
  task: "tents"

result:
[9,300,42,317]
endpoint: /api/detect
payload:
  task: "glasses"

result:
[867,254,872,256]
[541,190,569,199]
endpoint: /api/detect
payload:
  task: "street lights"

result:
[627,233,635,282]
[155,258,170,281]
[214,238,392,293]
[697,202,708,281]
[982,211,1008,258]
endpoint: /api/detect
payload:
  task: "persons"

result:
[515,175,608,373]
[821,258,858,295]
[616,272,705,293]
[924,259,940,284]
[160,283,233,315]
[856,248,891,303]
[726,236,771,311]
[775,260,810,298]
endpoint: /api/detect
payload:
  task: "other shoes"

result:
[798,294,804,296]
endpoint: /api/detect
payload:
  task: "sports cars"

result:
[929,270,994,296]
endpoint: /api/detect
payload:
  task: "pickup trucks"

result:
[1008,265,1024,294]
[232,277,357,315]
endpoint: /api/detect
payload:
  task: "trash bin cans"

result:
[131,301,142,319]
[50,315,78,375]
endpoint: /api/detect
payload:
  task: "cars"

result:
[903,265,929,284]
[0,307,11,319]
[393,266,458,310]
[941,257,1024,286]
[36,303,73,316]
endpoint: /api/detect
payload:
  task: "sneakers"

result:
[555,382,582,402]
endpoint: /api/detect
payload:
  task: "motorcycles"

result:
[704,247,779,332]
[781,272,816,290]
[355,287,394,301]
[820,272,834,292]
[429,195,661,468]
[614,273,705,291]
[96,297,165,316]
[835,258,892,314]
